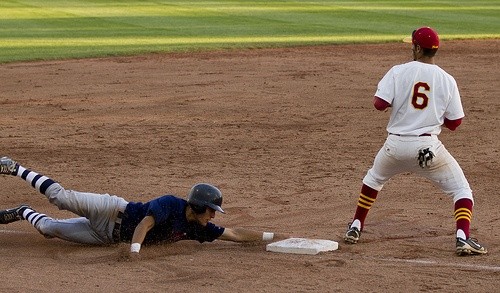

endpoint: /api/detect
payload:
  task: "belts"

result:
[113,211,123,243]
[389,133,431,136]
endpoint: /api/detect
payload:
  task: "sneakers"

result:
[344,222,361,244]
[0,205,32,224]
[0,157,17,175]
[456,237,487,255]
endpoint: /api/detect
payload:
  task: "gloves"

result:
[418,147,436,168]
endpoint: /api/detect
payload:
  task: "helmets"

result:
[187,184,226,214]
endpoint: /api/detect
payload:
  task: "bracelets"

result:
[130,243,141,253]
[263,232,274,241]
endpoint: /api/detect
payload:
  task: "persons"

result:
[0,157,303,257]
[344,27,488,255]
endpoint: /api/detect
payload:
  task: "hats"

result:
[412,27,439,50]
